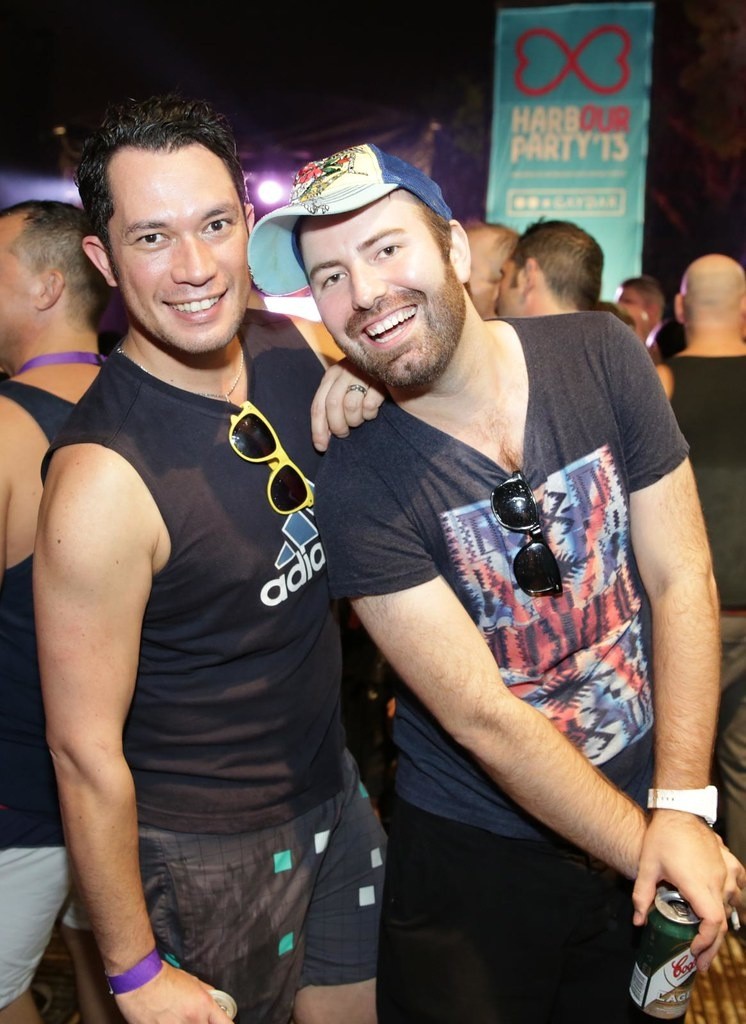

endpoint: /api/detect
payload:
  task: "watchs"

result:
[646,785,720,826]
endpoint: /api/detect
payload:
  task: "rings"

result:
[347,385,367,395]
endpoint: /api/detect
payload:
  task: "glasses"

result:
[491,470,563,596]
[229,401,314,515]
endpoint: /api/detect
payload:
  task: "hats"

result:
[246,142,453,296]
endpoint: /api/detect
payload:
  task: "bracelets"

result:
[100,948,165,995]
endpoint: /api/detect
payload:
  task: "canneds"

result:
[629,890,703,1020]
[207,989,238,1024]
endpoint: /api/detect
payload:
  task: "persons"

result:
[245,144,746,1023]
[494,219,605,319]
[0,197,115,1023]
[599,276,687,368]
[32,101,388,1024]
[455,221,521,317]
[655,252,746,934]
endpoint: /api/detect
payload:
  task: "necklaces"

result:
[116,347,244,405]
